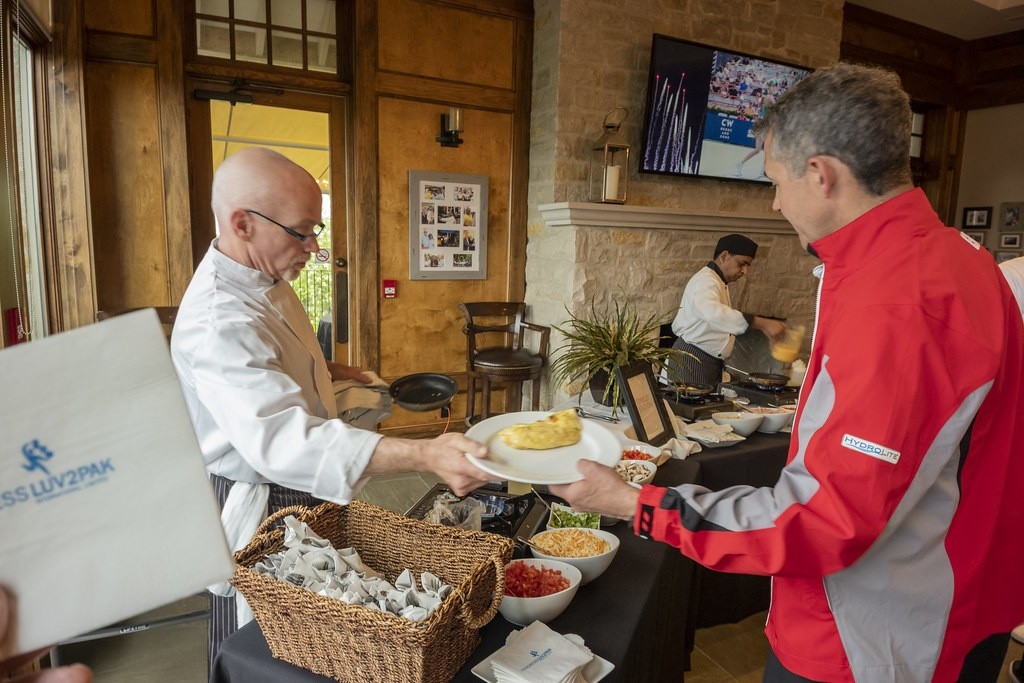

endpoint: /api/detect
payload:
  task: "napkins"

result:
[490,619,595,683]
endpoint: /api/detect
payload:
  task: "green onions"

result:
[549,506,601,530]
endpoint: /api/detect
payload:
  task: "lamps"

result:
[436,105,465,148]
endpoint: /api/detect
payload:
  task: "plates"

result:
[462,410,623,486]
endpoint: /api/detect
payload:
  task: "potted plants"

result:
[558,300,695,411]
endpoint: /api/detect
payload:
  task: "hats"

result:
[713,233,758,259]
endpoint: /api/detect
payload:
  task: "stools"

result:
[463,301,550,430]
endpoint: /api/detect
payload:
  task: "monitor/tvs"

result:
[637,33,816,186]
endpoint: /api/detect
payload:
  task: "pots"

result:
[724,363,791,387]
[667,380,713,396]
[365,371,459,412]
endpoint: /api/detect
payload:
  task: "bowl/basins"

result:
[711,411,765,437]
[742,407,796,435]
[530,527,620,586]
[622,444,662,464]
[613,460,657,486]
[779,403,797,411]
[601,480,643,527]
[546,502,600,530]
[493,558,582,627]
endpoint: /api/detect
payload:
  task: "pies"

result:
[498,409,583,449]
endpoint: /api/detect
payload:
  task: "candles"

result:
[602,163,620,200]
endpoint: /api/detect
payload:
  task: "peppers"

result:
[622,450,654,461]
[503,560,570,598]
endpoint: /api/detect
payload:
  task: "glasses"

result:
[246,210,326,245]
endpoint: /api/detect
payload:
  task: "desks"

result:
[572,384,805,628]
[207,456,699,683]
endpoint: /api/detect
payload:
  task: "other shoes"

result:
[760,167,765,176]
[736,160,744,177]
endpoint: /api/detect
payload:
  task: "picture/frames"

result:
[962,206,992,228]
[615,356,676,450]
[967,231,987,249]
[1000,233,1021,248]
[993,250,1021,265]
[999,203,1024,233]
[408,170,489,282]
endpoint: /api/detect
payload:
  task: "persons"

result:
[0,587,93,683]
[548,65,1024,683]
[711,57,797,177]
[170,146,507,683]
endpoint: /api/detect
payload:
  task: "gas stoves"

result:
[716,381,800,408]
[662,393,734,423]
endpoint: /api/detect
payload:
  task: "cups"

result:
[768,322,805,363]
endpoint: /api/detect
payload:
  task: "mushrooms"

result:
[613,462,651,483]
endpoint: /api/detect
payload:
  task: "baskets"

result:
[229,498,514,683]
